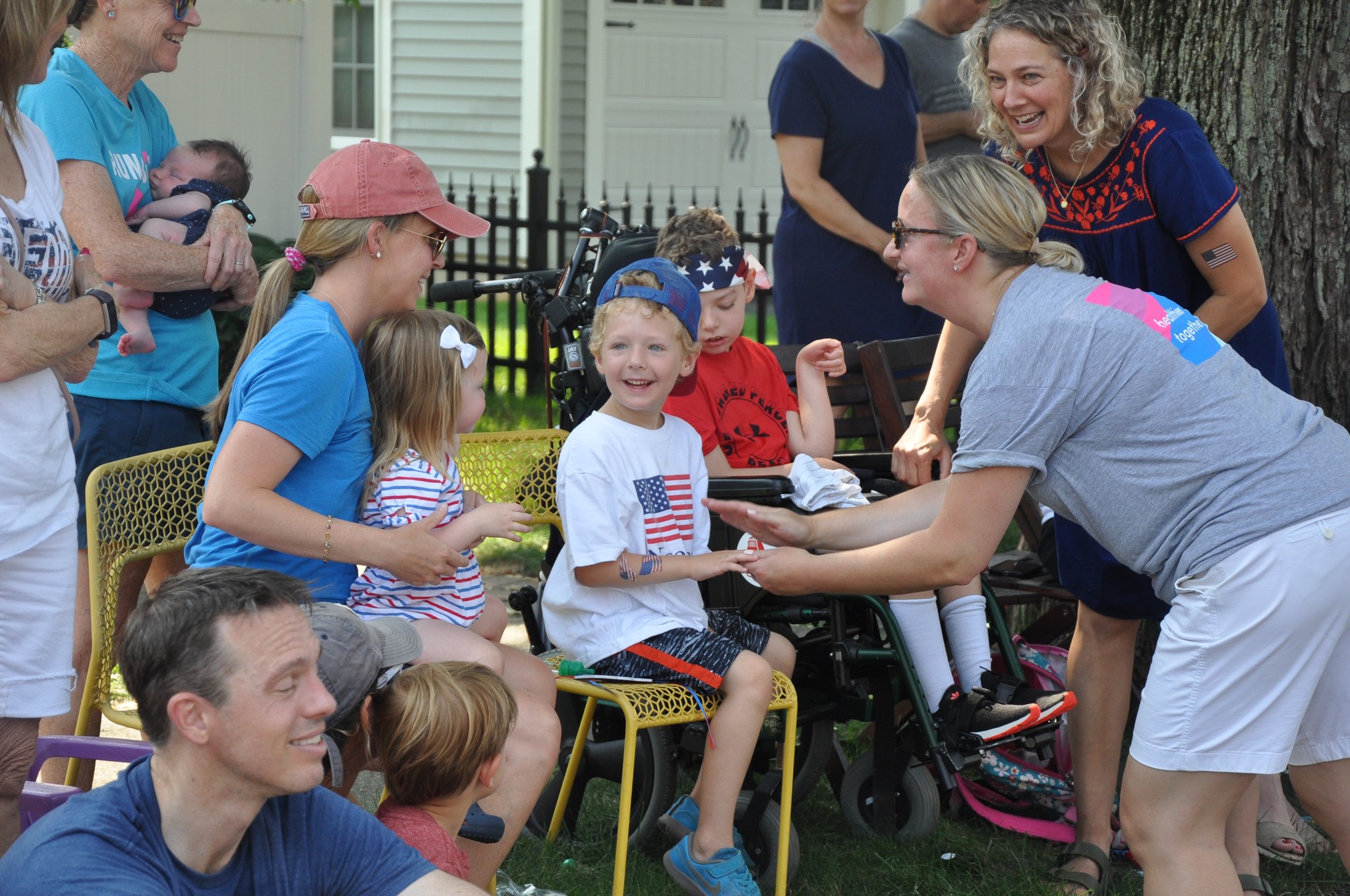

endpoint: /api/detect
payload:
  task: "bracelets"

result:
[79,247,91,256]
[32,281,47,304]
[323,514,332,564]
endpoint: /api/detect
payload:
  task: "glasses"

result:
[167,0,197,21]
[892,219,987,253]
[382,222,449,261]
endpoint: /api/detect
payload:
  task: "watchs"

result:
[77,288,118,348]
[212,198,256,229]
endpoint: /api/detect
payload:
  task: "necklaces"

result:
[1044,139,1092,208]
[988,264,1032,338]
[306,288,356,345]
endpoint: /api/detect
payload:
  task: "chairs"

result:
[12,334,1153,896]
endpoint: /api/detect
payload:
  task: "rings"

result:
[235,262,244,266]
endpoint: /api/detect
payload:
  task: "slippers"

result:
[1256,821,1307,866]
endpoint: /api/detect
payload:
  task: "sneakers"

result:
[663,831,762,896]
[656,794,757,878]
[931,684,1041,742]
[979,665,1077,728]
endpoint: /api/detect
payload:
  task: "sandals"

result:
[1237,874,1274,896]
[1043,841,1111,896]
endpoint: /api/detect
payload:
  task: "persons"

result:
[297,600,423,809]
[1,0,132,860]
[699,151,1350,896]
[11,0,259,795]
[658,209,1077,743]
[882,0,991,158]
[889,0,1308,896]
[372,661,517,883]
[347,311,537,679]
[0,565,492,896]
[766,0,934,453]
[182,136,563,896]
[540,257,799,896]
[103,139,254,356]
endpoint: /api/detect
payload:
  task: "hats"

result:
[297,139,491,238]
[595,257,701,396]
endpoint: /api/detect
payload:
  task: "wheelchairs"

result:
[427,196,1065,893]
